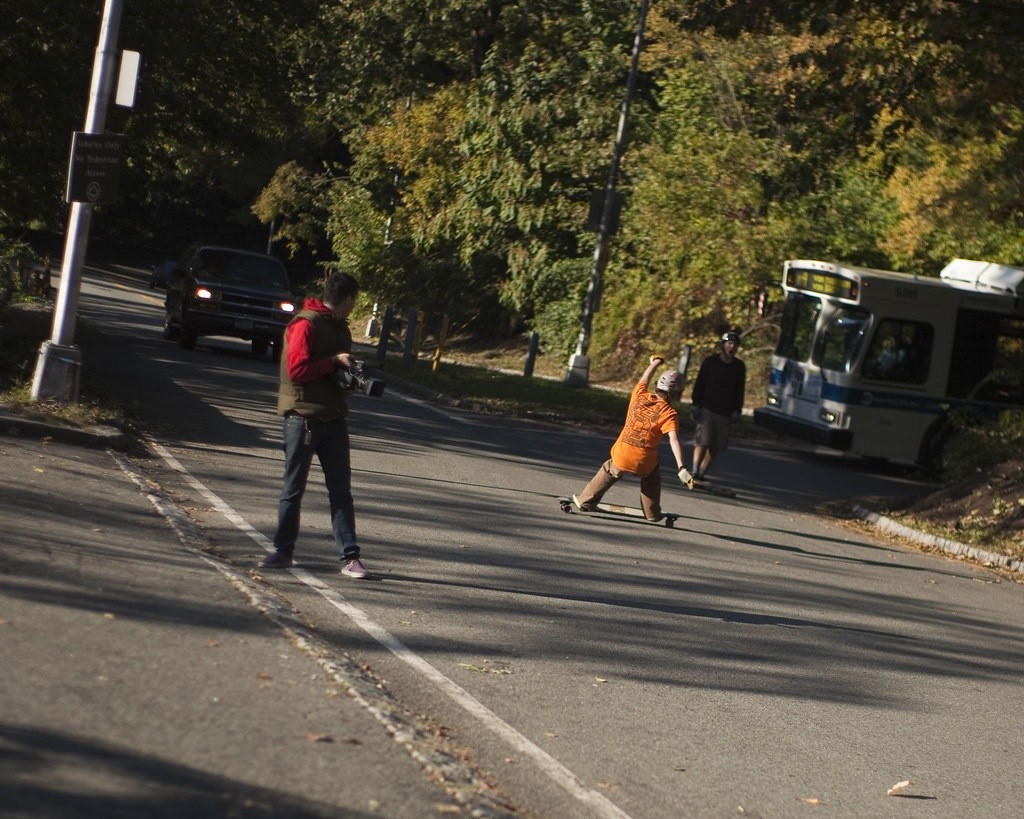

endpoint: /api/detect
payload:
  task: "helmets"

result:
[722,332,740,343]
[657,370,685,395]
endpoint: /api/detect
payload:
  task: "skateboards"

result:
[557,494,681,526]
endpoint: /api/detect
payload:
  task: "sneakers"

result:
[258,551,290,567]
[340,558,368,578]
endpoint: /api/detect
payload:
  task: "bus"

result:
[753,256,1024,485]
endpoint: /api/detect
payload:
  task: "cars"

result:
[149,254,181,288]
[162,245,307,365]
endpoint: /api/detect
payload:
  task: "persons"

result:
[883,334,906,372]
[572,355,694,522]
[691,332,746,485]
[258,273,372,579]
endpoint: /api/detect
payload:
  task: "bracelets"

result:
[680,465,687,471]
[653,357,662,361]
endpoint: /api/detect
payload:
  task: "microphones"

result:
[353,360,383,370]
